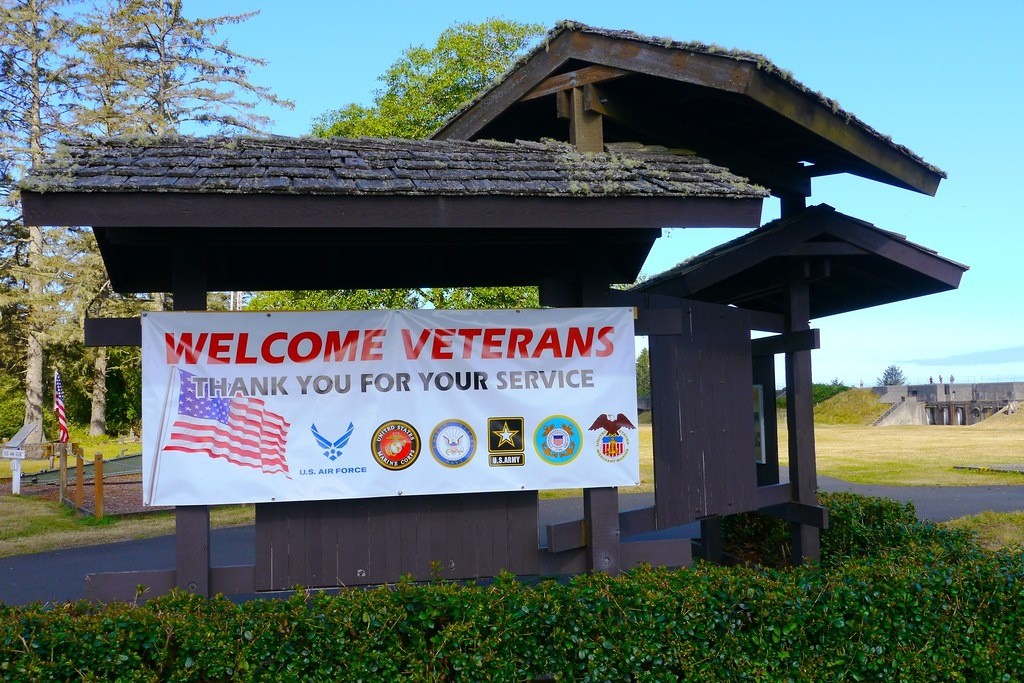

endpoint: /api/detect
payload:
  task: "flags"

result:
[54,373,69,442]
